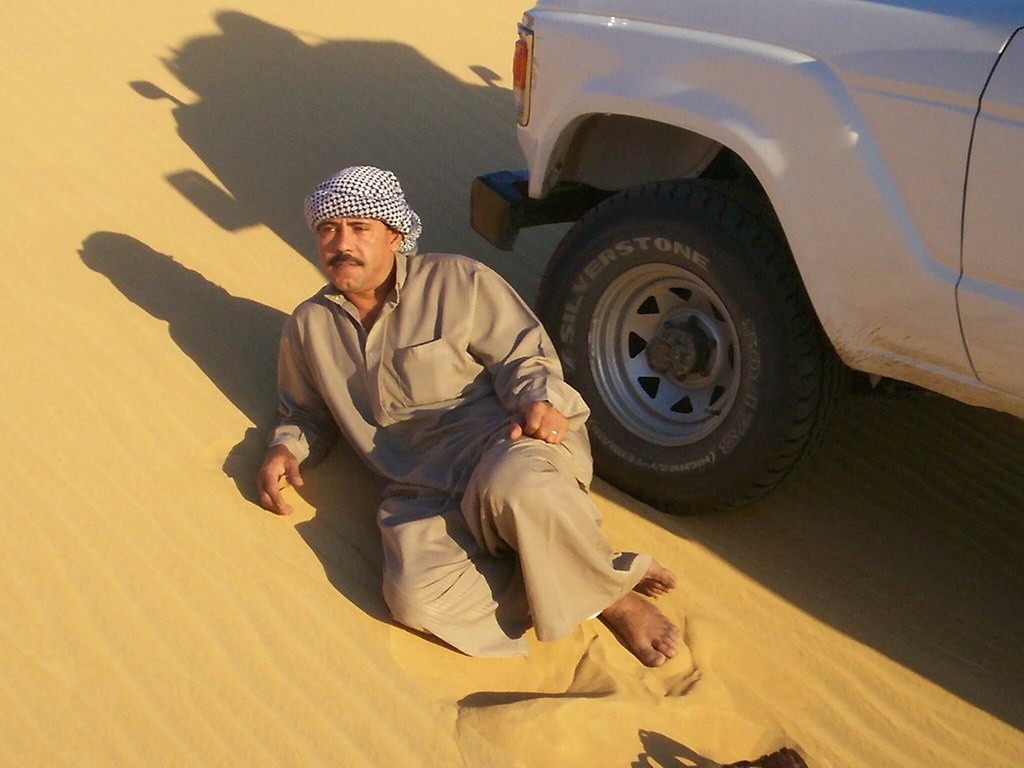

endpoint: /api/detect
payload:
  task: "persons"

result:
[257,165,680,666]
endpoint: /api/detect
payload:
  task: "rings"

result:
[552,431,558,435]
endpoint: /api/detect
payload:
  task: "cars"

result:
[471,0,1024,516]
